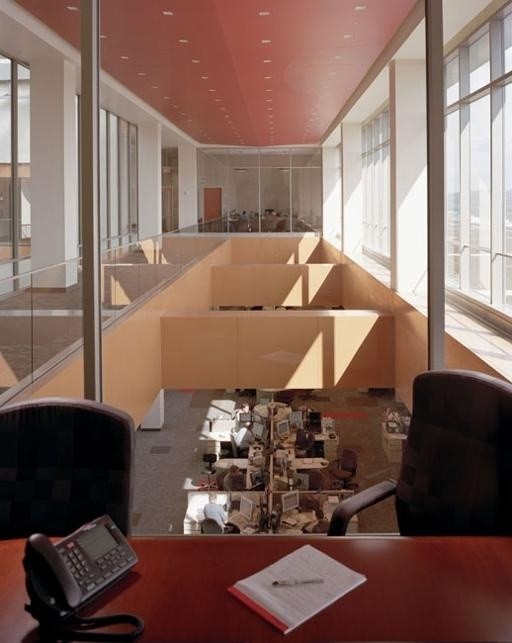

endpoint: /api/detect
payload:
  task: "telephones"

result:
[22,514,145,641]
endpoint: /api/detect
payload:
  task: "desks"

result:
[1,535,511,642]
[381,422,408,463]
[183,502,268,534]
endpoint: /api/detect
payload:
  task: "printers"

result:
[323,501,339,515]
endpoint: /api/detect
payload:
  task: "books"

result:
[226,544,368,635]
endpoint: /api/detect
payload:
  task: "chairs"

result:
[201,408,357,534]
[0,398,134,540]
[225,214,287,232]
[328,369,512,536]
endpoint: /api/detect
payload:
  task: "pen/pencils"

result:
[272,578,324,587]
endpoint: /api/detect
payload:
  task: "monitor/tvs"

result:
[294,474,309,489]
[239,496,255,520]
[252,422,264,437]
[282,490,299,514]
[277,419,290,438]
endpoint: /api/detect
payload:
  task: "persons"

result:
[312,509,330,533]
[222,465,248,491]
[235,403,251,432]
[271,504,281,534]
[203,494,228,528]
[291,424,307,459]
[230,209,283,232]
[236,422,255,457]
